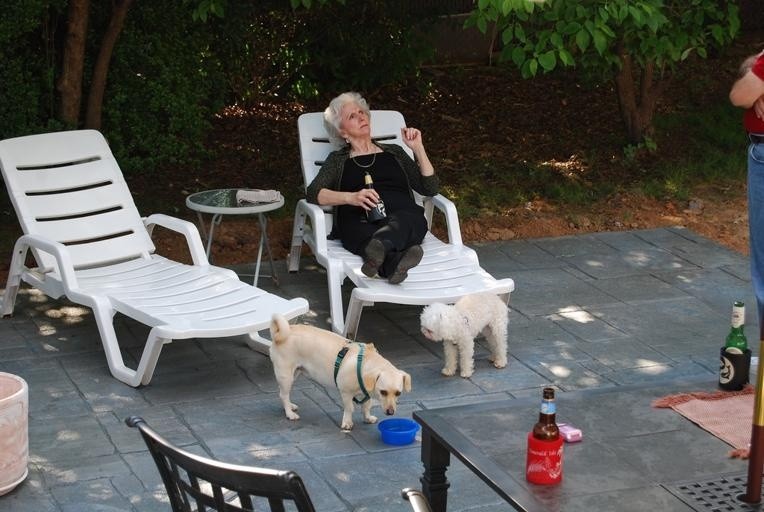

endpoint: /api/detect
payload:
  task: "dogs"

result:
[268,312,413,431]
[419,292,511,379]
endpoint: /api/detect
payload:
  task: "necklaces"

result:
[347,142,378,168]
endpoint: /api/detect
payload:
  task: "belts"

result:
[748,132,764,145]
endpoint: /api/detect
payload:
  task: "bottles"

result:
[524,386,566,488]
[718,300,751,386]
[362,171,386,222]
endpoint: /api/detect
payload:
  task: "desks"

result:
[183,184,285,294]
[406,375,761,511]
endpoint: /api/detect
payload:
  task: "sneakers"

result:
[361,236,387,279]
[385,245,424,285]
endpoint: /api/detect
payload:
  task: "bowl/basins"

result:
[378,418,418,446]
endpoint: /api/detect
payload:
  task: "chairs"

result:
[398,485,431,512]
[2,128,312,391]
[288,108,517,352]
[124,410,317,512]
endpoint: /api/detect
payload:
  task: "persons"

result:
[306,91,440,283]
[727,48,764,338]
